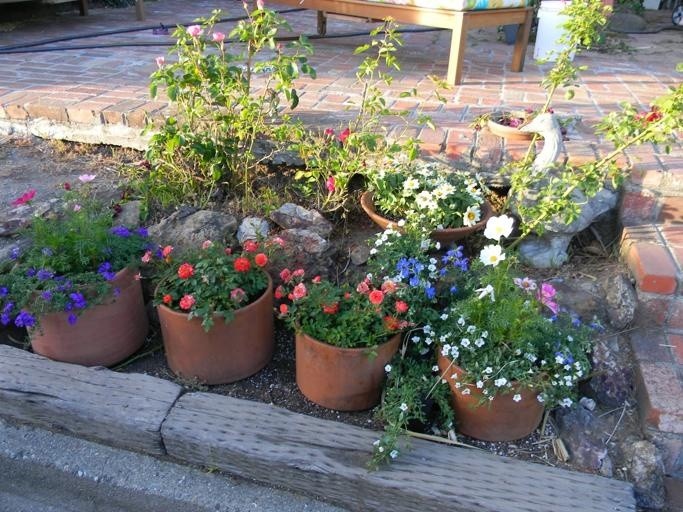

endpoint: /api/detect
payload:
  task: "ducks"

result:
[519,112,620,239]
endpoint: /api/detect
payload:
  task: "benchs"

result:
[253,0,534,86]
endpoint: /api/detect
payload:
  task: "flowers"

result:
[0,154,607,474]
